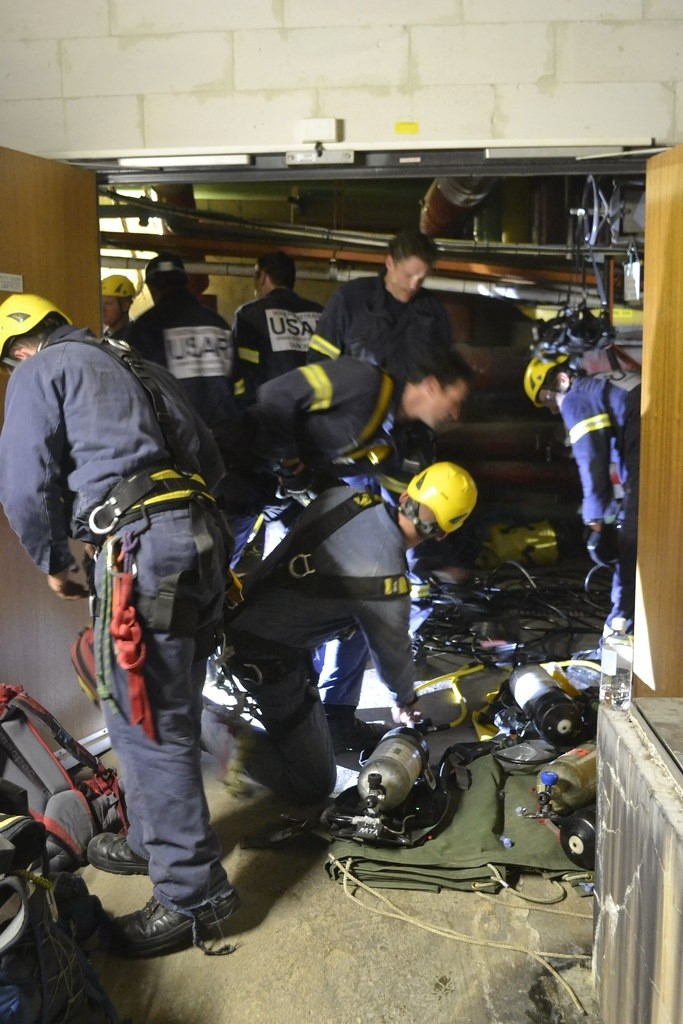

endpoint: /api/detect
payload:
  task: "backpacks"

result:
[0,683,132,876]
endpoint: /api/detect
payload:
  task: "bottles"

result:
[598,616,634,711]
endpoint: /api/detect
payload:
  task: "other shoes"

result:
[326,718,392,755]
[224,725,264,799]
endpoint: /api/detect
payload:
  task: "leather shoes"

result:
[99,889,243,957]
[86,833,150,876]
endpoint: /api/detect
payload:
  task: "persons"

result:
[109,253,239,507]
[257,347,473,755]
[223,461,477,804]
[305,225,454,665]
[231,250,324,533]
[101,275,136,337]
[0,293,236,957]
[523,351,641,666]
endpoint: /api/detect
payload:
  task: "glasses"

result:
[545,387,554,409]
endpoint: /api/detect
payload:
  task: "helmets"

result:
[145,254,188,283]
[101,275,135,298]
[523,351,569,408]
[0,294,73,358]
[408,462,477,533]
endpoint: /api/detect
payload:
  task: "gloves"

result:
[278,468,318,493]
